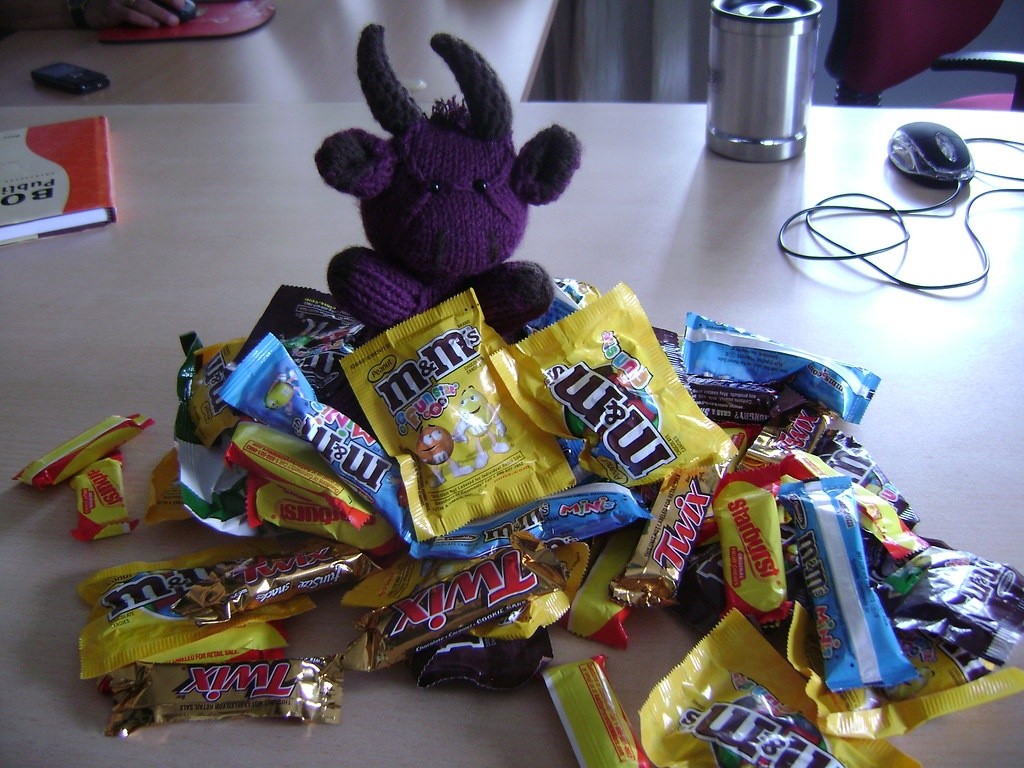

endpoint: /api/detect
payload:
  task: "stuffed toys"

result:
[313,24,582,332]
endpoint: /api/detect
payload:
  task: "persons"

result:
[0,0,185,41]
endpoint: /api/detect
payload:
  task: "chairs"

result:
[822,0,1024,111]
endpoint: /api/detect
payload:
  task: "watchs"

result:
[67,0,91,30]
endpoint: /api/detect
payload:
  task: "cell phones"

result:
[30,63,111,95]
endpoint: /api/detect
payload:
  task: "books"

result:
[0,116,117,247]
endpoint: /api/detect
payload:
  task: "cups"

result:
[704,0,823,163]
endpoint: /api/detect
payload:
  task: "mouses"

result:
[888,121,976,189]
[150,0,197,24]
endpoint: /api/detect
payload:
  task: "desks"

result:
[0,101,1024,768]
